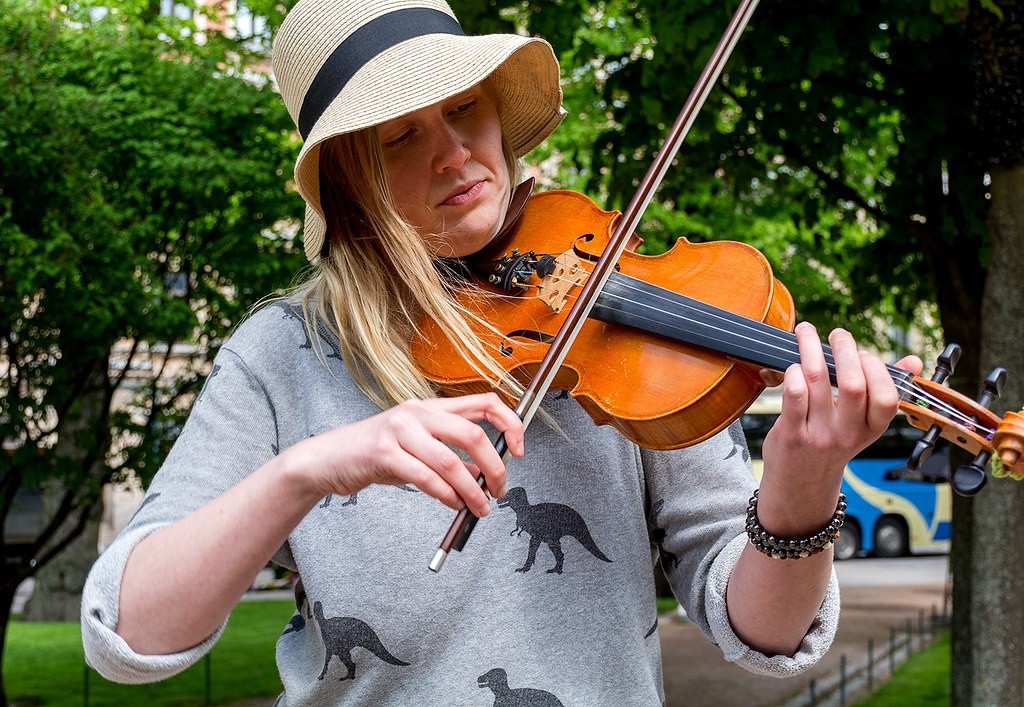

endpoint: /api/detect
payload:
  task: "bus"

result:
[739,397,954,560]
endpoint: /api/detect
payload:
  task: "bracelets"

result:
[747,487,850,559]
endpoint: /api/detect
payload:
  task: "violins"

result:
[409,189,1024,498]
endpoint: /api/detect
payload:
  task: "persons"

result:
[79,0,925,706]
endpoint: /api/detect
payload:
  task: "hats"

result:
[271,0,567,261]
